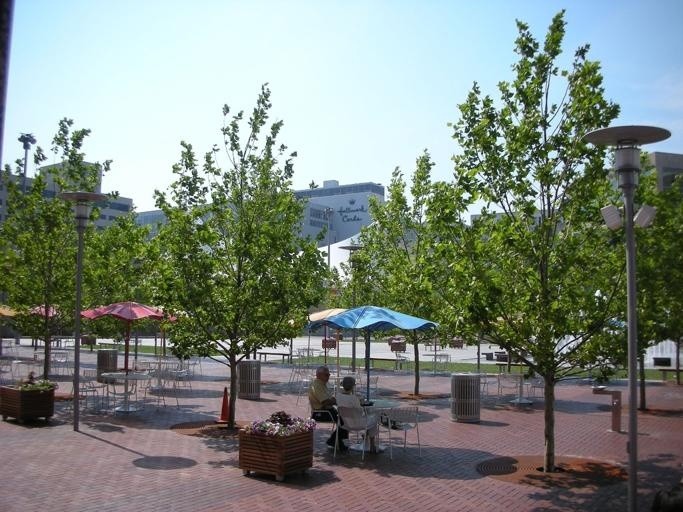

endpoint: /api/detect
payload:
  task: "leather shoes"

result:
[326,439,348,451]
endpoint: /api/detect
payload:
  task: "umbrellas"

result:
[0,301,61,318]
[81,300,177,370]
[285,306,439,402]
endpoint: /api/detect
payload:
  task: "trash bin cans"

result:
[238,361,261,400]
[97,350,118,384]
[451,373,481,424]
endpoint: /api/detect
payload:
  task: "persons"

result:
[309,366,349,452]
[336,377,383,453]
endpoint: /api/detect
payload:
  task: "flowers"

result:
[242,411,316,437]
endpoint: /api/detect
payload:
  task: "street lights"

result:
[57,189,109,432]
[339,245,363,372]
[579,126,671,511]
[323,206,333,340]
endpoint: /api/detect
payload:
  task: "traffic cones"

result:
[214,386,234,425]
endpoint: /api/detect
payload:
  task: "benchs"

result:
[250,351,293,362]
[481,351,508,362]
[657,366,682,381]
[422,354,451,365]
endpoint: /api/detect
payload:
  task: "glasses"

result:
[352,384,356,386]
[325,373,331,376]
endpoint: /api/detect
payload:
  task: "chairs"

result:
[306,374,421,464]
[478,371,546,407]
[0,340,203,418]
[287,347,379,406]
